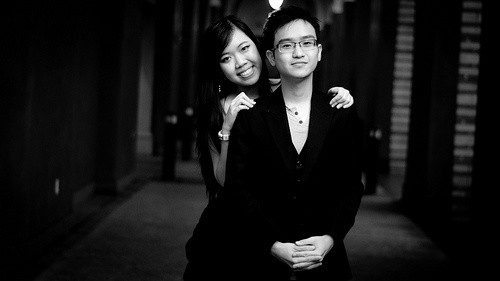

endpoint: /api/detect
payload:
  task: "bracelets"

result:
[217,130,229,141]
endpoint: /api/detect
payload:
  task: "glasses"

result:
[273,39,317,53]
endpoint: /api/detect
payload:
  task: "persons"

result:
[218,5,366,281]
[182,15,355,281]
